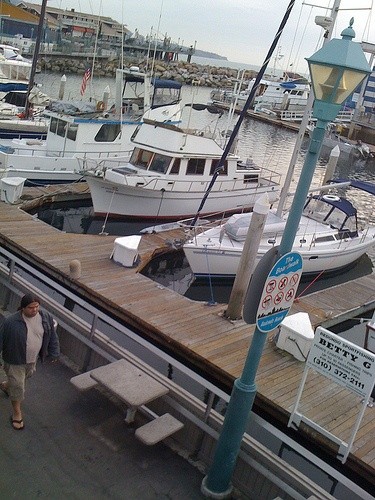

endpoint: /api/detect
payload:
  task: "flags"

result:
[80,64,91,96]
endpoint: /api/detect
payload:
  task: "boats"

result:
[0,1,284,226]
[180,177,375,282]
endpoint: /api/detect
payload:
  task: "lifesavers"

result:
[96,100,105,112]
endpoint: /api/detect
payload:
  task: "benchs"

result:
[70,369,97,391]
[135,413,184,447]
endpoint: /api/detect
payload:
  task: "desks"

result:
[90,358,169,422]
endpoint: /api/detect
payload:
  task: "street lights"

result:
[199,15,370,500]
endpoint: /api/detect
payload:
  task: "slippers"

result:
[0,383,9,397]
[10,414,24,430]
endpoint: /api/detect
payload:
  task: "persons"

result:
[0,293,60,430]
